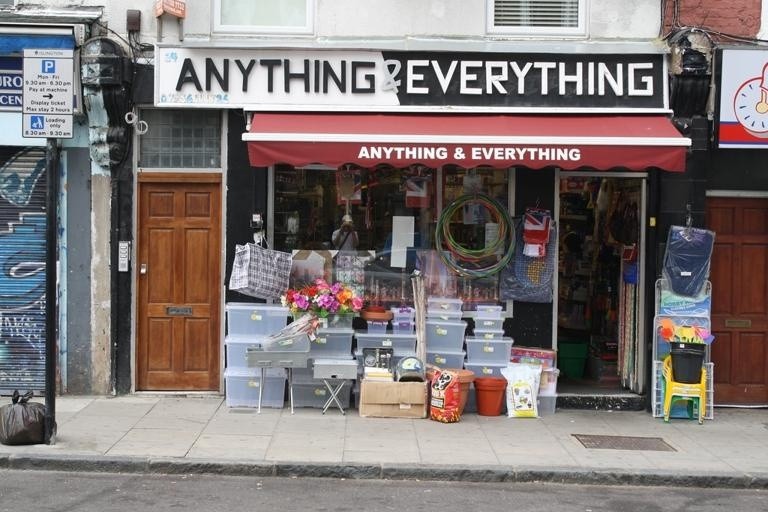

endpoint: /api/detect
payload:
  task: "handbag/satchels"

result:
[229,242,292,299]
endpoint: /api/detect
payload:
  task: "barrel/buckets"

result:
[396,356,425,382]
[668,341,706,383]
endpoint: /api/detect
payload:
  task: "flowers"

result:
[279,276,367,322]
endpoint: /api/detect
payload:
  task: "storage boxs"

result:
[392,320,415,335]
[288,380,351,409]
[427,298,463,311]
[354,349,416,376]
[473,329,504,340]
[359,376,428,419]
[463,360,509,377]
[511,345,556,367]
[427,311,462,323]
[366,320,387,334]
[473,317,505,329]
[558,342,617,381]
[424,320,467,353]
[307,328,354,360]
[225,301,290,340]
[289,355,353,384]
[475,302,503,318]
[223,367,287,409]
[538,367,559,395]
[355,329,418,355]
[426,352,465,371]
[465,336,513,364]
[536,396,557,415]
[390,306,416,322]
[224,336,262,370]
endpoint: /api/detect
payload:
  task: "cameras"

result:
[343,223,350,232]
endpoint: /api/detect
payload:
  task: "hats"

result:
[342,215,352,221]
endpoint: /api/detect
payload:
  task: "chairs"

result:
[662,355,709,425]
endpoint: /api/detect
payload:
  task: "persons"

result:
[332,214,359,250]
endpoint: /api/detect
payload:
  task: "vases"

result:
[670,342,706,384]
[427,369,474,415]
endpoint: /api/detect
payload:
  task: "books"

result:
[365,366,395,382]
